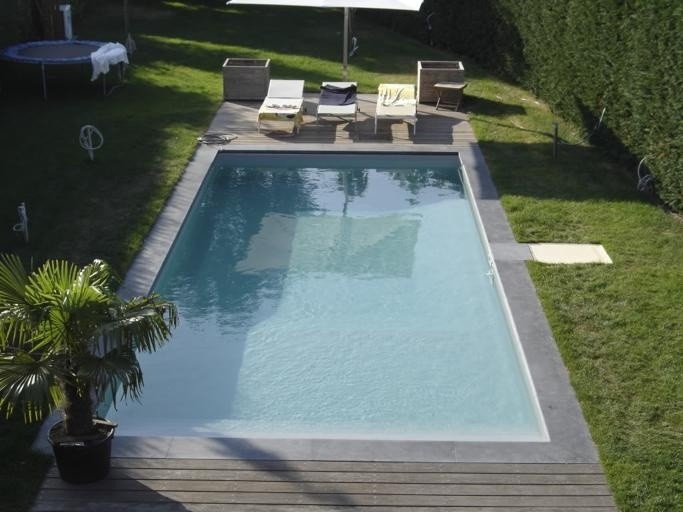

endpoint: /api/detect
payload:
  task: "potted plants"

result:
[0,255,178,484]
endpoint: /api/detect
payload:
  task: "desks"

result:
[434,81,468,112]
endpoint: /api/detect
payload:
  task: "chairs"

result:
[256,80,305,135]
[317,82,360,133]
[374,83,418,135]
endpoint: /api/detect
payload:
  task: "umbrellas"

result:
[225,1,426,81]
